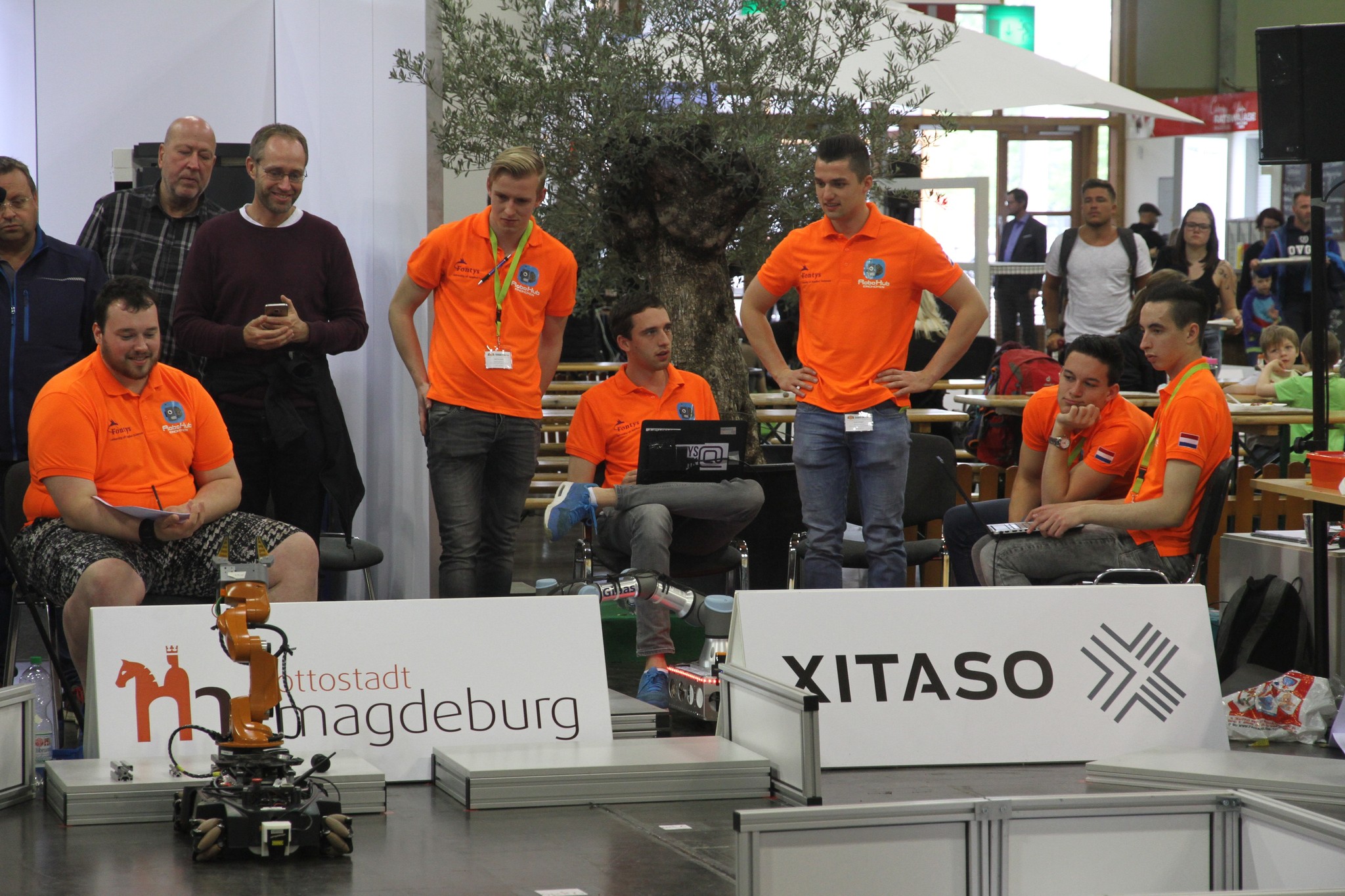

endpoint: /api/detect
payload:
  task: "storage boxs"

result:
[1307,450,1345,491]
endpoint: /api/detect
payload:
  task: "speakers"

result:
[1254,22,1345,164]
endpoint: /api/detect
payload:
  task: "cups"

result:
[1303,513,1330,547]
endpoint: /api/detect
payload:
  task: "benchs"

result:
[522,362,625,507]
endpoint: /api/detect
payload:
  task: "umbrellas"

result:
[620,0,1205,124]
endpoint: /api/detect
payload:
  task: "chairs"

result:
[785,434,957,590]
[1080,455,1238,585]
[573,521,748,597]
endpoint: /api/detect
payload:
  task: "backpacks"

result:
[1214,574,1313,683]
[962,341,1062,467]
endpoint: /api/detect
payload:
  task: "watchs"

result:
[1045,328,1059,337]
[1049,433,1071,449]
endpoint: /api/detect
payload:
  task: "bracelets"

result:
[140,514,160,548]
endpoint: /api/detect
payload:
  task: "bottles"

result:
[20,657,53,787]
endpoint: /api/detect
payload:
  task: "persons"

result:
[173,123,370,544]
[0,156,110,678]
[10,277,319,696]
[740,133,1345,588]
[544,292,765,710]
[388,145,578,598]
[76,114,228,370]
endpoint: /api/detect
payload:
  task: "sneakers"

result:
[543,480,601,543]
[635,666,672,712]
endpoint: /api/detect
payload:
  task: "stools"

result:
[319,533,384,600]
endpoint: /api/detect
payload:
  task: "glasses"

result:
[0,195,33,213]
[1184,222,1211,231]
[257,161,307,183]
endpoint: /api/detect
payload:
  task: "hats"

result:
[1138,203,1161,215]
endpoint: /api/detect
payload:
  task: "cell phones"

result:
[265,303,288,317]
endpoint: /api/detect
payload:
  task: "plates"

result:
[1236,403,1288,409]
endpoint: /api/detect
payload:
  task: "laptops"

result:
[635,420,747,486]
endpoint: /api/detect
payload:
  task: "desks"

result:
[954,393,1160,417]
[750,392,797,405]
[930,379,986,391]
[1230,411,1345,477]
[754,409,970,443]
[1218,478,1345,678]
